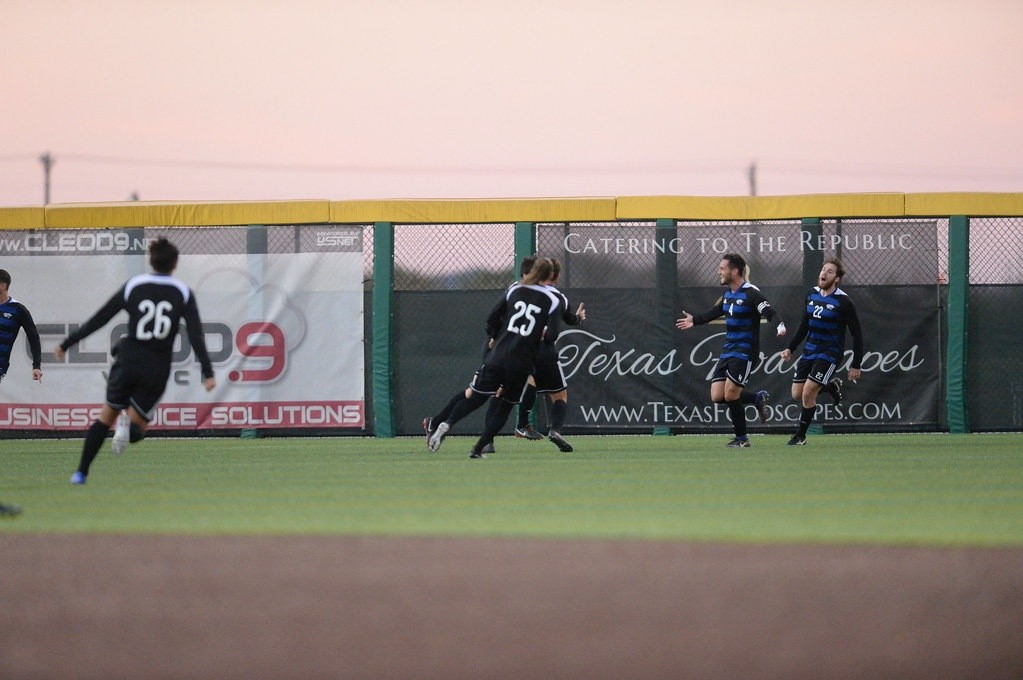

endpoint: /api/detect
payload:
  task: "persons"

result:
[781,260,863,444]
[676,254,786,447]
[0,269,42,385]
[56,239,216,484]
[423,257,585,458]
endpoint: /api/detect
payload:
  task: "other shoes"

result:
[71,472,85,484]
[111,409,130,455]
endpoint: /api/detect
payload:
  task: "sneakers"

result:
[786,434,806,445]
[726,438,750,447]
[423,417,432,445]
[469,447,482,459]
[428,422,451,452]
[830,377,844,406]
[515,423,544,441]
[548,430,573,452]
[481,443,495,453]
[756,392,767,422]
